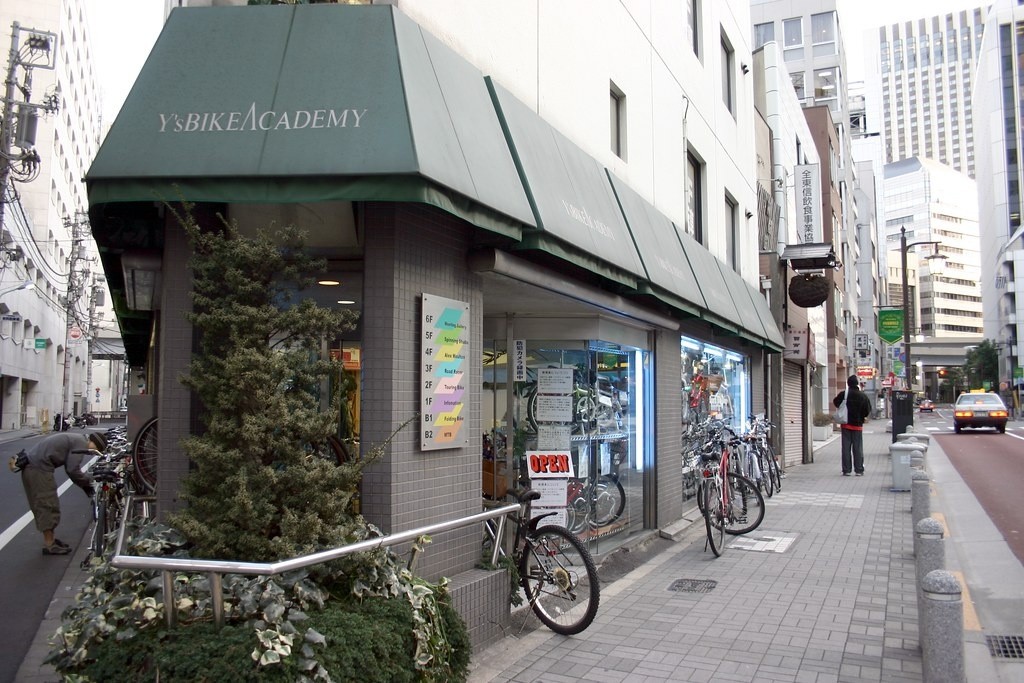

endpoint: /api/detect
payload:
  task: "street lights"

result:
[967,366,977,390]
[994,335,1015,417]
[900,223,949,389]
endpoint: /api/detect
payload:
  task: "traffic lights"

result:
[938,369,945,378]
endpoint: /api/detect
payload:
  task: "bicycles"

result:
[483,488,601,636]
[71,425,142,569]
[681,412,784,558]
[485,362,626,540]
[131,413,355,506]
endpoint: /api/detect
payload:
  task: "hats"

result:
[90,433,107,455]
[848,375,859,387]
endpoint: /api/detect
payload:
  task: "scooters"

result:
[53,408,99,432]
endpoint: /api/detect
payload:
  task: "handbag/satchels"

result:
[831,389,848,424]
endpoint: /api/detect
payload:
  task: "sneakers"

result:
[55,539,71,552]
[43,543,67,555]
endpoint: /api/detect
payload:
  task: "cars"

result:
[952,393,1008,434]
[916,397,935,412]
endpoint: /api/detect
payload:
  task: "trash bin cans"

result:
[891,388,914,443]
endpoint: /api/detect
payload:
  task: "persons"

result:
[833,375,872,475]
[21,432,107,555]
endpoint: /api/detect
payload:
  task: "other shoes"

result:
[844,471,851,475]
[856,472,863,475]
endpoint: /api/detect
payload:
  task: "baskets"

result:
[681,434,712,472]
